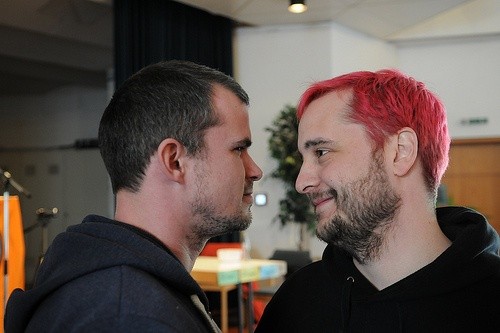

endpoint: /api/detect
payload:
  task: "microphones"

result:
[37,208,58,217]
[0,171,31,198]
[74,138,99,150]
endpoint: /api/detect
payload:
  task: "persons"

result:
[254,71,500,333]
[4,61,263,332]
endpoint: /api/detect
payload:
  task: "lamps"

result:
[288,0,308,15]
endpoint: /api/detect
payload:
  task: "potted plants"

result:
[265,103,318,252]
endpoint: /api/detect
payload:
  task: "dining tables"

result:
[191,254,288,333]
